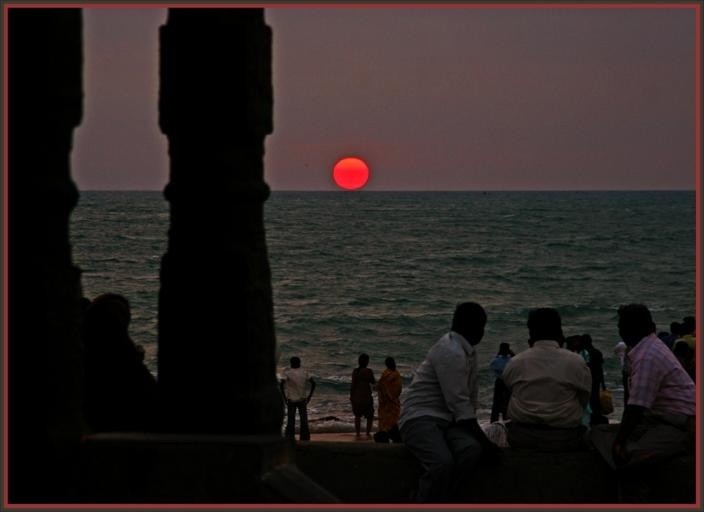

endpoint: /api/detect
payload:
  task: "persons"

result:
[373,355,404,440]
[78,290,197,432]
[500,306,593,428]
[398,302,513,502]
[587,300,695,502]
[488,315,695,428]
[279,357,315,441]
[349,354,375,440]
